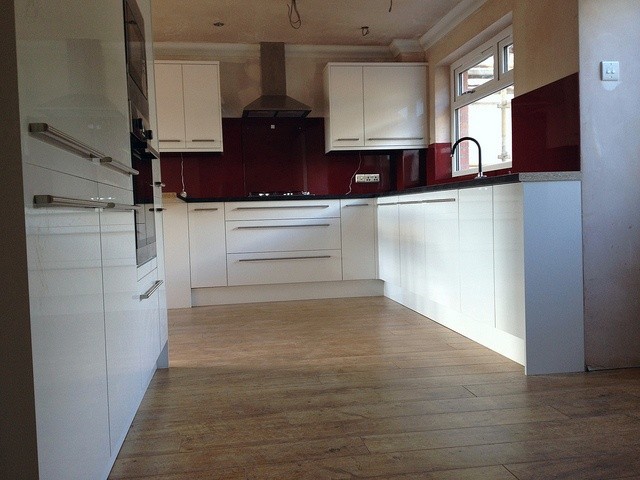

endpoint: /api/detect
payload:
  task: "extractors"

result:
[241,42,312,122]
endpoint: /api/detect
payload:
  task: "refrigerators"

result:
[9,1,140,479]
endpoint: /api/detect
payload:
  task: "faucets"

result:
[450,136,484,177]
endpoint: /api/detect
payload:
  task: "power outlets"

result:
[355,174,379,182]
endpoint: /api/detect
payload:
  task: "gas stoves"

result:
[247,190,318,199]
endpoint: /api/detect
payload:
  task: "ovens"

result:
[127,0,171,396]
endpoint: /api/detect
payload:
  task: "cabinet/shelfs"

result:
[492,171,584,376]
[421,180,459,332]
[137,254,163,397]
[323,63,364,154]
[99,182,142,471]
[2,0,135,191]
[341,197,378,299]
[182,60,224,152]
[2,163,110,478]
[162,192,191,311]
[376,194,398,302]
[187,202,227,307]
[364,62,430,150]
[397,187,422,313]
[225,199,342,299]
[458,178,492,353]
[153,58,184,152]
